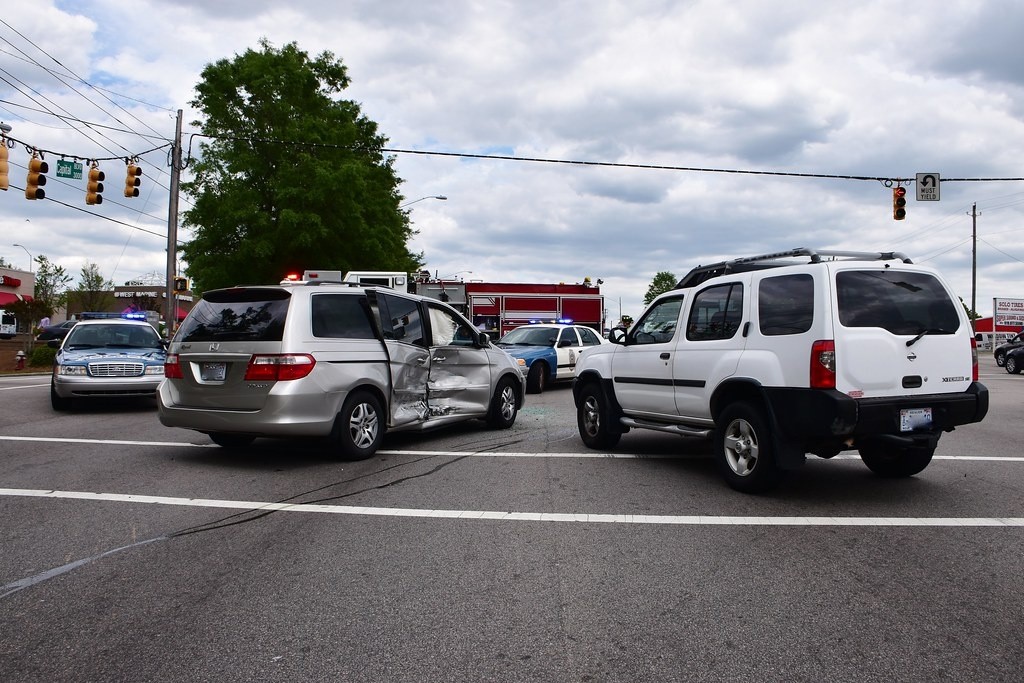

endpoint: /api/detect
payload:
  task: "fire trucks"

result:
[280,270,605,338]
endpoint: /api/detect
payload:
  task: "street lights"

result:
[13,244,32,273]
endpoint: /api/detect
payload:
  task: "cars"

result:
[38,320,77,340]
[48,317,171,409]
[994,329,1024,374]
[495,324,609,395]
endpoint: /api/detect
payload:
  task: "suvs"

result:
[571,246,990,495]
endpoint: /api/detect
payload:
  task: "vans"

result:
[975,332,1021,352]
[0,310,16,339]
[156,283,527,462]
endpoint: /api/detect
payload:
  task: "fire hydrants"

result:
[15,351,27,370]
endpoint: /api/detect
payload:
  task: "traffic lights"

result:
[25,158,49,200]
[173,277,190,295]
[893,187,906,220]
[86,169,105,205]
[124,165,142,197]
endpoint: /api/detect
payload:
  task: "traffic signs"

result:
[916,173,940,201]
